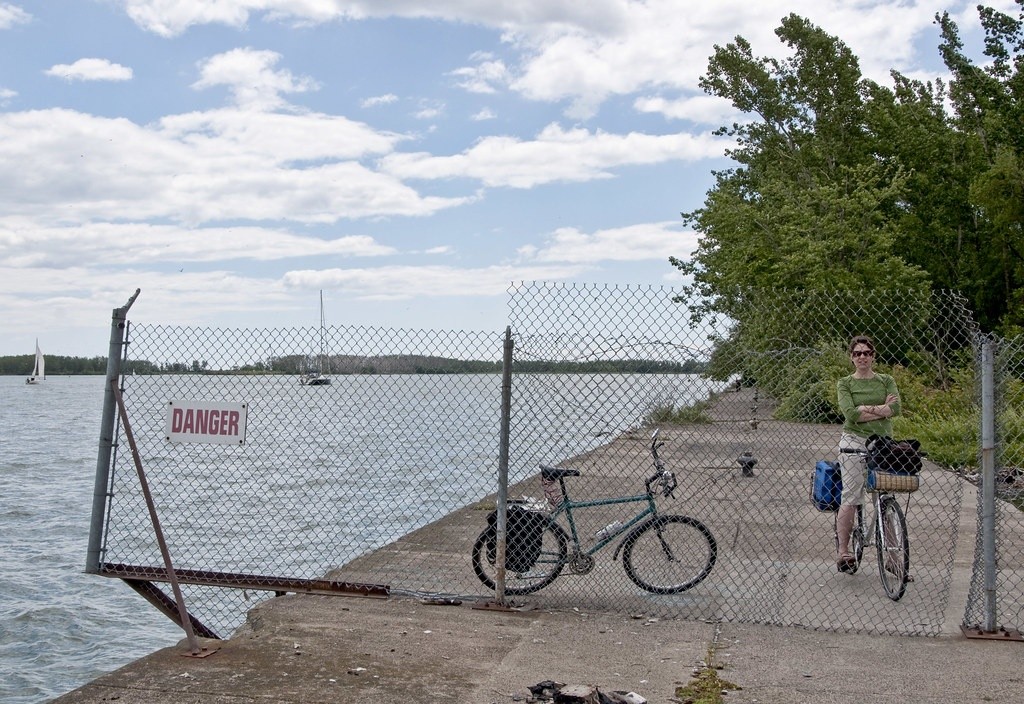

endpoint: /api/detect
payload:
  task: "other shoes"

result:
[837,552,856,572]
[885,560,915,582]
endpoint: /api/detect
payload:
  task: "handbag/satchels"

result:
[865,434,922,474]
[486,505,543,573]
[812,460,844,512]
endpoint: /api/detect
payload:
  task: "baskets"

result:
[860,455,919,494]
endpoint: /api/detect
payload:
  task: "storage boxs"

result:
[816,459,842,507]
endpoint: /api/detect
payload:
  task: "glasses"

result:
[852,350,873,357]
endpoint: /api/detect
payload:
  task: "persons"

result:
[837,336,915,583]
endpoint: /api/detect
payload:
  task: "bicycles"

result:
[472,425,717,596]
[809,447,928,602]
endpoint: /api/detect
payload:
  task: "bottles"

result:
[596,520,624,541]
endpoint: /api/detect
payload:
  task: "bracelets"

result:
[871,405,876,413]
